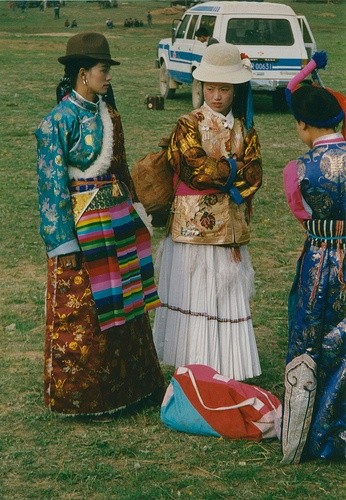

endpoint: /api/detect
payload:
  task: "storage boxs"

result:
[144,94,164,110]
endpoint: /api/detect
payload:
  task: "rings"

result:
[65,262,73,268]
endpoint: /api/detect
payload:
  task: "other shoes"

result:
[79,415,113,422]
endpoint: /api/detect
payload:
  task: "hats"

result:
[192,43,252,84]
[58,32,120,65]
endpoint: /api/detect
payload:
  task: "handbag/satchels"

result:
[159,364,283,442]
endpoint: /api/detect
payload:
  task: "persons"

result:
[8,1,201,28]
[195,28,219,47]
[35,32,164,422]
[277,85,346,467]
[153,44,261,380]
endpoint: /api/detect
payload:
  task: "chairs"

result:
[201,25,260,45]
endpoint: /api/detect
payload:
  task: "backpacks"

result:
[131,113,201,214]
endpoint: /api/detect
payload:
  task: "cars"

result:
[155,1,316,113]
